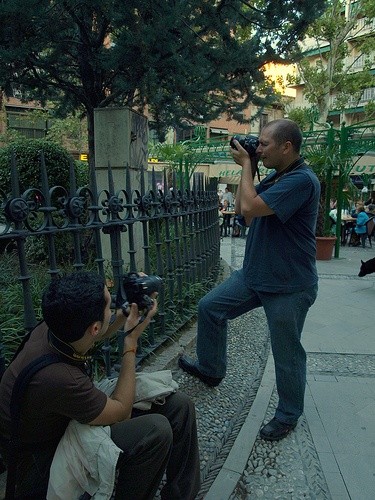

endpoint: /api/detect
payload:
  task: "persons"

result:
[178,120,320,440]
[0,270,201,500]
[329,199,375,246]
[218,188,250,236]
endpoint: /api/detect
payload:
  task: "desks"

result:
[221,211,236,237]
[341,217,357,246]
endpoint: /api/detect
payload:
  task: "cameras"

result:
[230,135,259,158]
[123,272,163,311]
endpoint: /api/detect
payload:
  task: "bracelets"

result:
[123,349,136,356]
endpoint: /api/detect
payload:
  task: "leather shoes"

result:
[178,355,223,387]
[260,415,291,440]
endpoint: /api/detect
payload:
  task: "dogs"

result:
[358,256,375,278]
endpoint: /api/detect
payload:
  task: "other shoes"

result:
[341,237,361,247]
[232,229,240,237]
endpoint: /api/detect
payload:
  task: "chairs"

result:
[358,216,375,248]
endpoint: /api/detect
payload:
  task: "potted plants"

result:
[304,145,338,260]
